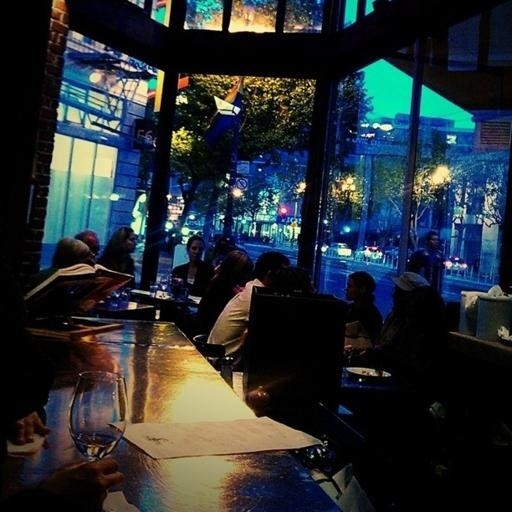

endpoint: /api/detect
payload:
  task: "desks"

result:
[88,286,402,439]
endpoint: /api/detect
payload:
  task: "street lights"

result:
[292,182,306,201]
[341,177,357,242]
[212,92,242,242]
[233,187,243,234]
[428,163,453,253]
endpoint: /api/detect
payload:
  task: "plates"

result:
[342,364,391,378]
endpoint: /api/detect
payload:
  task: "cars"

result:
[318,241,472,280]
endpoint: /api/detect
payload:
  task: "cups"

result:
[66,368,128,463]
[109,288,121,307]
[121,288,132,306]
[149,280,158,299]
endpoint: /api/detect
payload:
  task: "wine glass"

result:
[159,275,168,298]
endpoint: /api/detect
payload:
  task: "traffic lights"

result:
[280,205,288,219]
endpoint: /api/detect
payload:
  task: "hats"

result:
[392,272,431,292]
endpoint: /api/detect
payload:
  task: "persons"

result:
[383,232,444,373]
[172,235,315,370]
[344,271,384,333]
[1,227,135,512]
